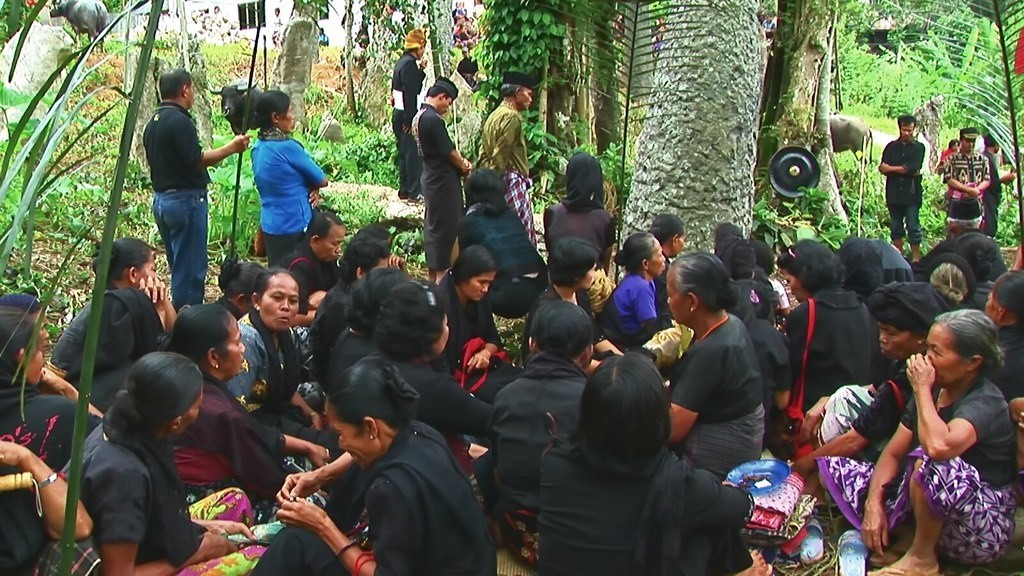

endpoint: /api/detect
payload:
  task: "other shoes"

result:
[399,191,408,198]
[408,194,426,205]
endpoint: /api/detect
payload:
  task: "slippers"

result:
[799,519,824,563]
[838,530,868,576]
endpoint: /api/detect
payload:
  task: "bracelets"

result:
[309,412,318,417]
[336,540,357,557]
[38,471,64,489]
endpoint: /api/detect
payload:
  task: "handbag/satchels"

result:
[767,298,814,461]
[455,337,516,393]
[334,509,370,555]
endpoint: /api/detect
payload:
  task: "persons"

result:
[935,139,961,175]
[980,133,1016,237]
[943,127,991,238]
[143,67,253,313]
[449,0,488,88]
[0,154,1024,576]
[412,75,473,285]
[211,6,329,48]
[879,115,925,261]
[761,15,776,40]
[251,90,328,266]
[387,28,428,206]
[477,71,537,250]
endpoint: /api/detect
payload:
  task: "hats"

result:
[405,28,427,49]
[960,127,979,139]
[503,71,535,90]
[0,294,41,314]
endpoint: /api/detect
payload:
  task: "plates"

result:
[726,460,790,492]
